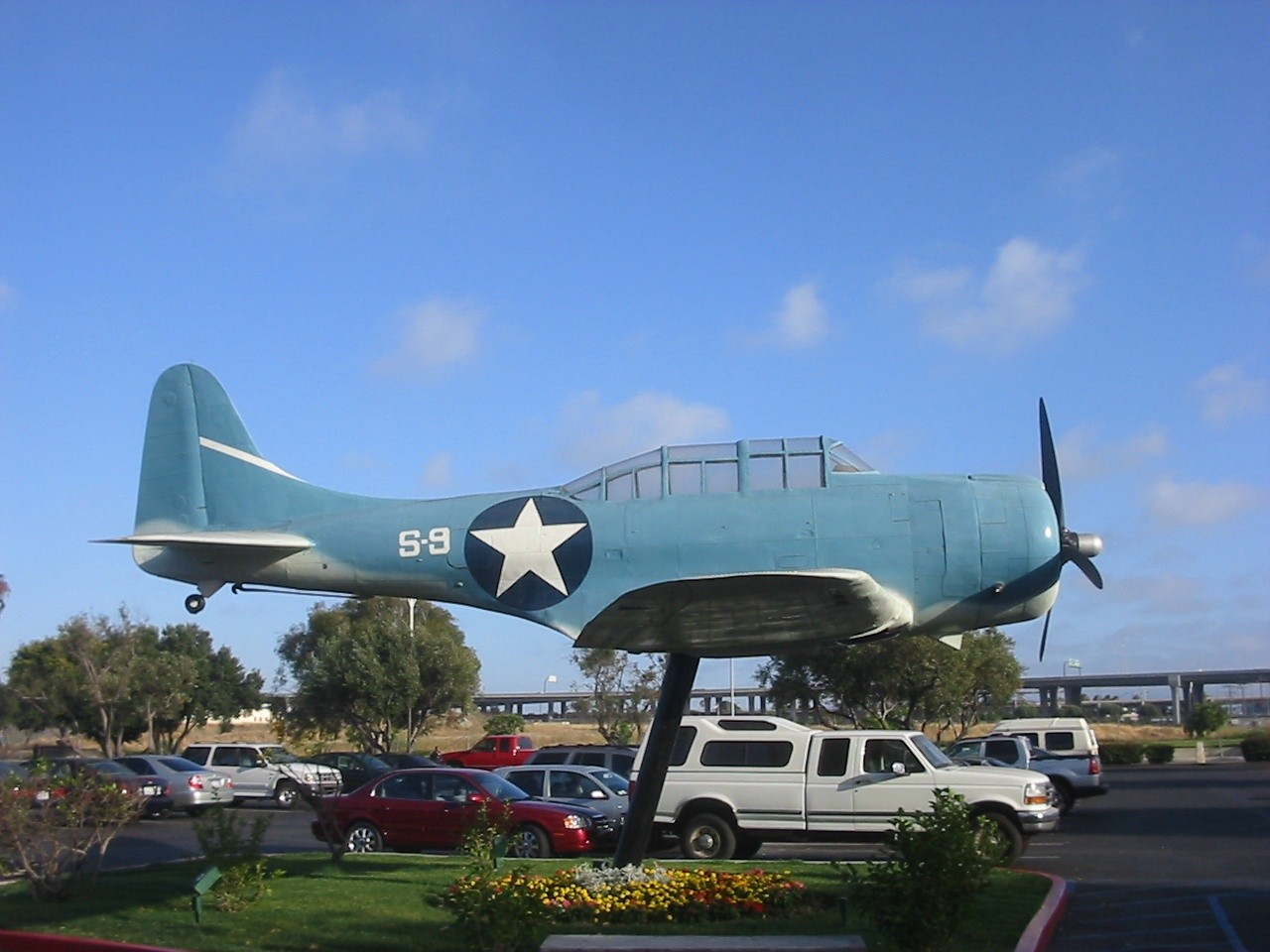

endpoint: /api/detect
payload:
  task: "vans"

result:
[981,716,1100,756]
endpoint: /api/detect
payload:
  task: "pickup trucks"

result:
[626,715,1061,866]
[940,736,1109,815]
[440,734,538,769]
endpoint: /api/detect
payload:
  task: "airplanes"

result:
[86,364,1105,664]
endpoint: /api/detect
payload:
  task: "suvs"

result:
[524,744,639,777]
[179,741,344,809]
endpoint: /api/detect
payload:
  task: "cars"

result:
[0,761,75,813]
[21,758,173,819]
[310,768,613,858]
[906,755,1015,767]
[491,764,630,828]
[115,755,233,818]
[308,751,450,791]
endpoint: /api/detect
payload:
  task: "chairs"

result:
[393,779,415,796]
